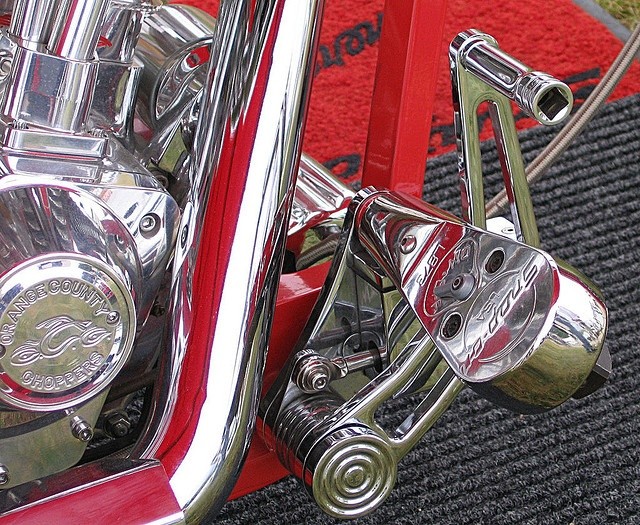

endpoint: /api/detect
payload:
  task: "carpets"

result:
[210,94,640,525]
[147,1,639,191]
